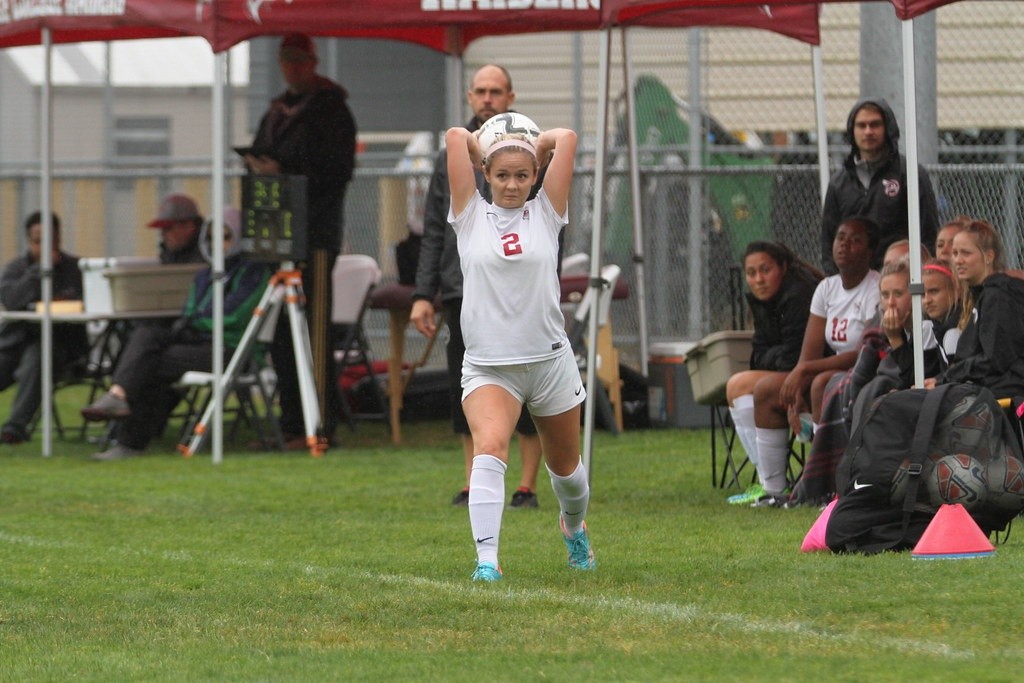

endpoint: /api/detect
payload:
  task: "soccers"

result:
[472,110,542,162]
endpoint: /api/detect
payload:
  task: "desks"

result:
[0,309,183,441]
[370,277,621,444]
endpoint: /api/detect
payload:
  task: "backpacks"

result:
[826,385,1024,557]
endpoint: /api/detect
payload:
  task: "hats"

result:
[147,194,200,231]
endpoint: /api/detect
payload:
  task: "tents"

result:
[0,0,962,459]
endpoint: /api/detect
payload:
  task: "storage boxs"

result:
[683,331,754,405]
[103,264,209,311]
[648,342,728,428]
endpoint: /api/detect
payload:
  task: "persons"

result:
[444,112,597,579]
[146,195,210,263]
[0,211,87,444]
[245,31,356,451]
[645,97,1024,554]
[410,64,565,508]
[80,206,272,462]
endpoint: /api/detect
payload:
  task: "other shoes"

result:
[82,393,131,421]
[0,422,30,445]
[92,439,148,461]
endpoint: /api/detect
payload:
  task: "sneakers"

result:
[751,486,791,509]
[452,487,469,507]
[725,482,790,506]
[558,509,595,571]
[506,485,539,512]
[470,560,502,584]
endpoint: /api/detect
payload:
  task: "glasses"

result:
[208,232,231,241]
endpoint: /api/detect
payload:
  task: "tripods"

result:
[182,261,331,459]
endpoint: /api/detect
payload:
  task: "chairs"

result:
[560,253,621,435]
[24,254,393,456]
